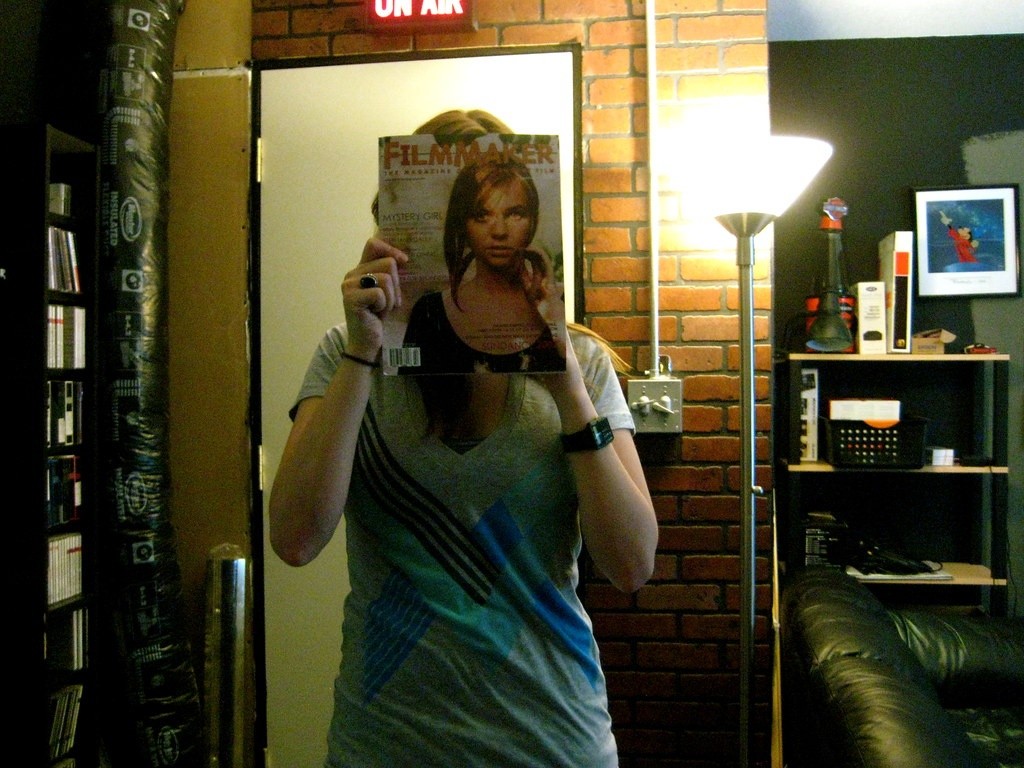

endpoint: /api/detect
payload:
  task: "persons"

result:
[269,111,659,768]
[397,151,565,375]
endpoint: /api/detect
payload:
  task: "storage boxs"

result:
[846,281,888,355]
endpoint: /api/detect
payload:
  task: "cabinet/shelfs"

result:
[782,350,1014,634]
[0,117,122,768]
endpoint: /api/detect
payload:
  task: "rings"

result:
[360,273,378,289]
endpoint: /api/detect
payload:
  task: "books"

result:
[380,135,565,375]
[46,608,89,671]
[45,455,81,530]
[45,380,82,449]
[45,684,83,759]
[46,226,80,294]
[49,182,72,216]
[46,303,85,370]
[46,532,82,606]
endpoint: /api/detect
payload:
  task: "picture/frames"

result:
[908,182,1022,299]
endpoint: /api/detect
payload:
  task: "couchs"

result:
[786,567,1024,768]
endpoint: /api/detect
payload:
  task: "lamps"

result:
[676,136,836,768]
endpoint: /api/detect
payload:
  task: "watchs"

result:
[559,416,614,452]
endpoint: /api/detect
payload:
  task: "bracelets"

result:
[340,352,379,369]
